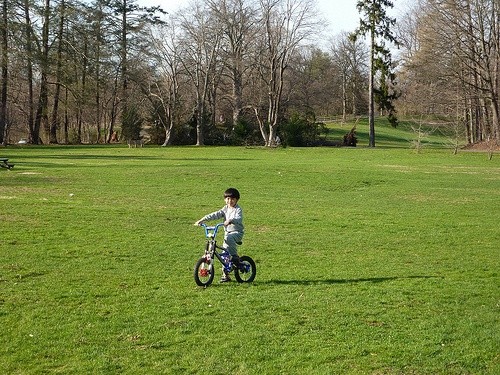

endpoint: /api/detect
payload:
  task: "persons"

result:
[194,188,244,283]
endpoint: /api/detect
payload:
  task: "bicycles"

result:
[194,222,256,285]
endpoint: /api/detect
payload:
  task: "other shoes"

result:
[219,276,230,283]
[229,256,238,264]
